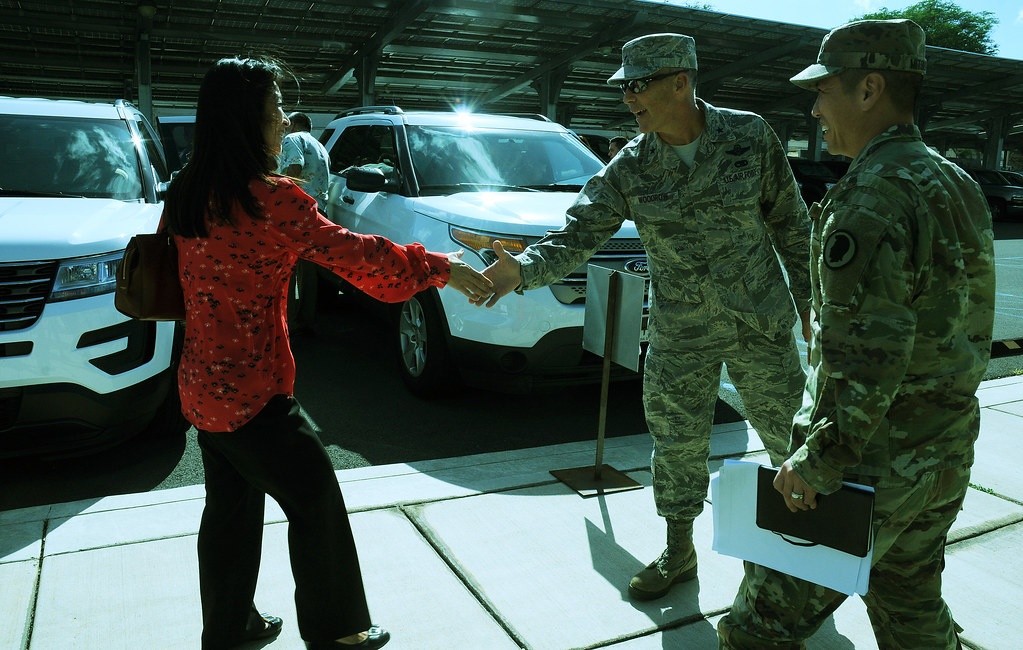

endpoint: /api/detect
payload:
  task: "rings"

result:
[791,492,804,500]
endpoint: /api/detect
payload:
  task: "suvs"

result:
[292,103,657,405]
[0,95,180,456]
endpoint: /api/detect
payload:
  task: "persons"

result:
[280,111,332,341]
[607,136,628,160]
[717,17,995,650]
[152,59,494,650]
[470,33,812,602]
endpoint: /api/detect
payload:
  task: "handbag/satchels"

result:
[115,224,186,321]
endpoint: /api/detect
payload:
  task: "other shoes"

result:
[294,326,317,337]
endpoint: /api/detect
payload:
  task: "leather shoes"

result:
[304,624,390,650]
[201,612,283,649]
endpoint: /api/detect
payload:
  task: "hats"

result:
[789,19,928,92]
[607,33,698,85]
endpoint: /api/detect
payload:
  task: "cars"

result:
[821,161,851,177]
[788,157,840,205]
[976,169,1023,220]
[576,134,614,164]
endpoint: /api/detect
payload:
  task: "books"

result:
[755,464,875,558]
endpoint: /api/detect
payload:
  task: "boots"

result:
[628,518,697,601]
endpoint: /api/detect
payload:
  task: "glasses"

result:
[619,69,689,94]
